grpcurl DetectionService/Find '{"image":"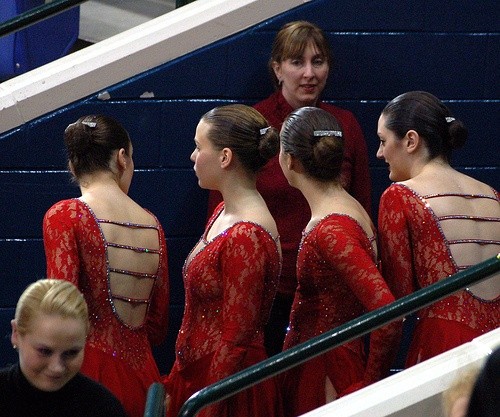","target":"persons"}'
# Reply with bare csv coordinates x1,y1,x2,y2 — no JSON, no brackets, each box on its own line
377,90,500,373
0,279,127,417
43,115,169,417
163,105,283,417
278,106,406,417
207,21,371,349
